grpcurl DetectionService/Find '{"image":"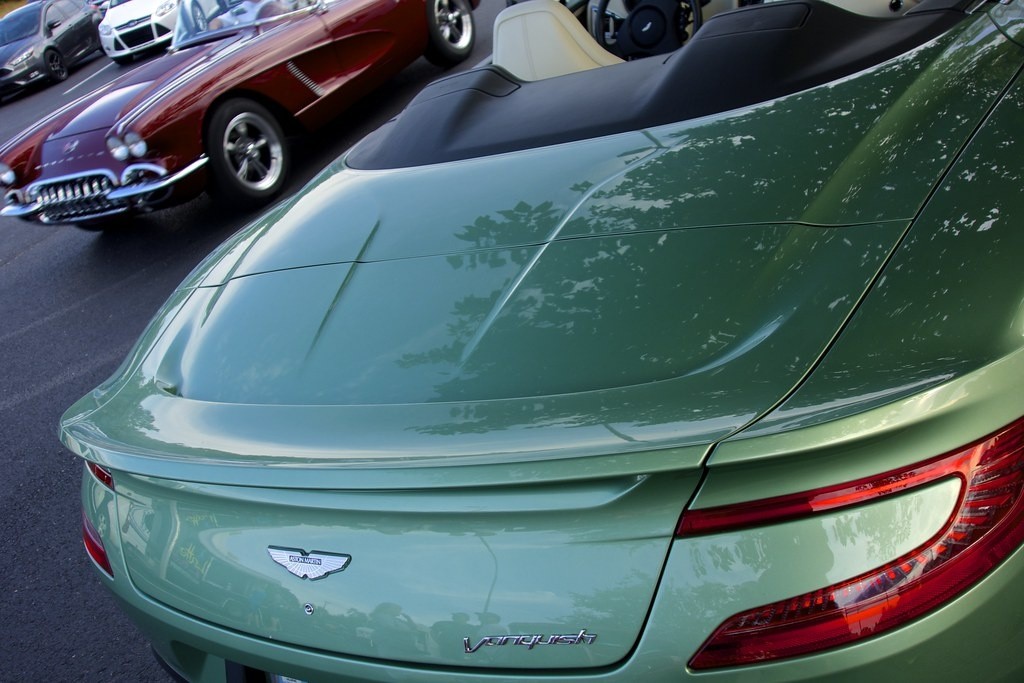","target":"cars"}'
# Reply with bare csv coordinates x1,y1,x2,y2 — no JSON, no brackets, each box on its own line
0,0,479,234
97,0,223,68
54,0,1021,681
0,0,103,102
